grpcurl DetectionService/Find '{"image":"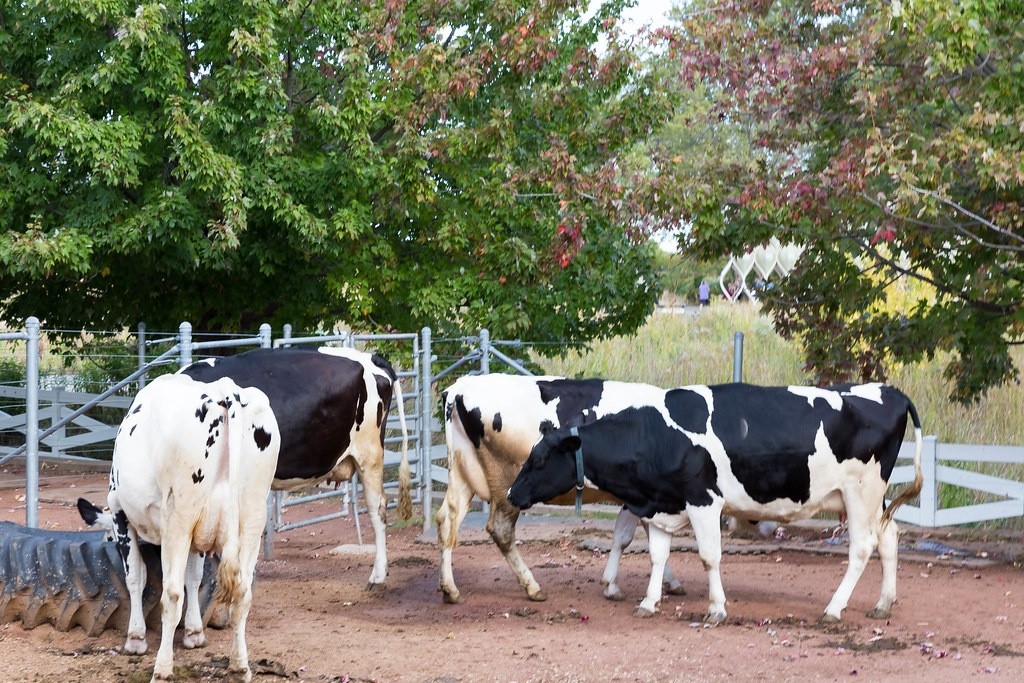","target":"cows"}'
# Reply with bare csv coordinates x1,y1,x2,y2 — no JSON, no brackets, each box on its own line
76,344,925,683
